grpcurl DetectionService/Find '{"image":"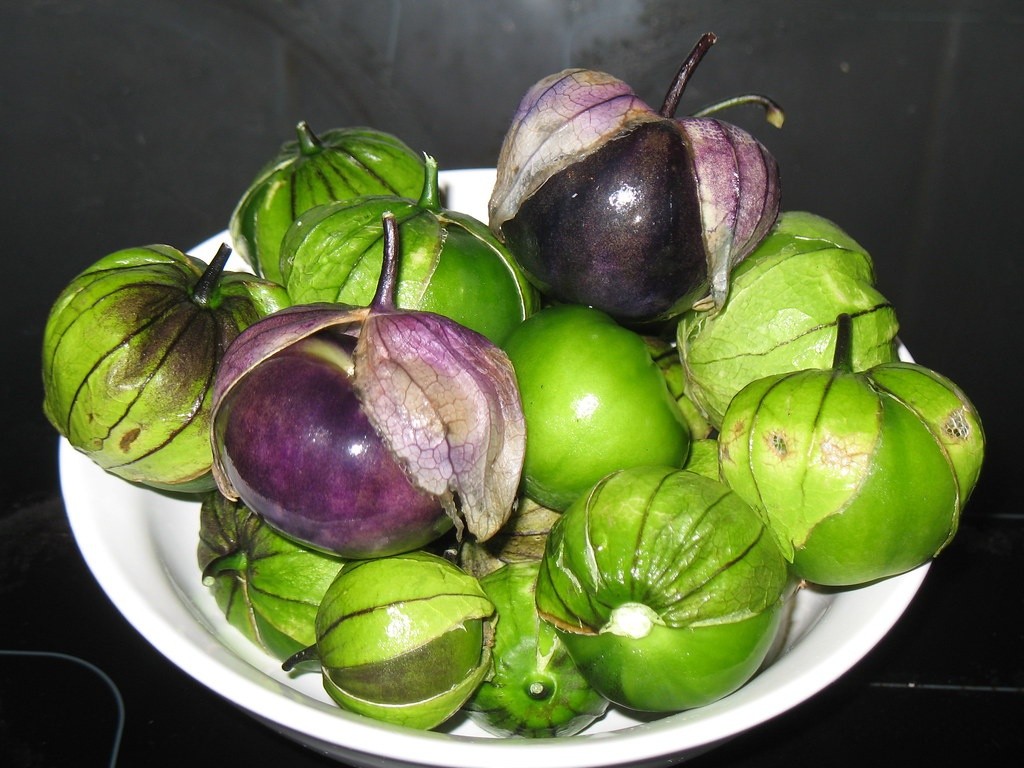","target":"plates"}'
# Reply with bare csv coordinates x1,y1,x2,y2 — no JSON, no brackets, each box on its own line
57,169,932,768
